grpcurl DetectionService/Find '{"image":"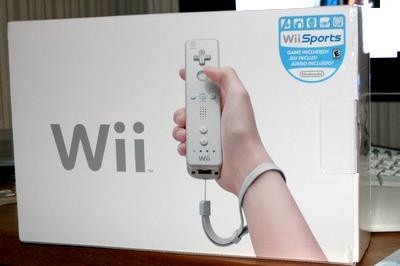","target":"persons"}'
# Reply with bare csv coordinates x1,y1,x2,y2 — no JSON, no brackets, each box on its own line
172,65,329,262
